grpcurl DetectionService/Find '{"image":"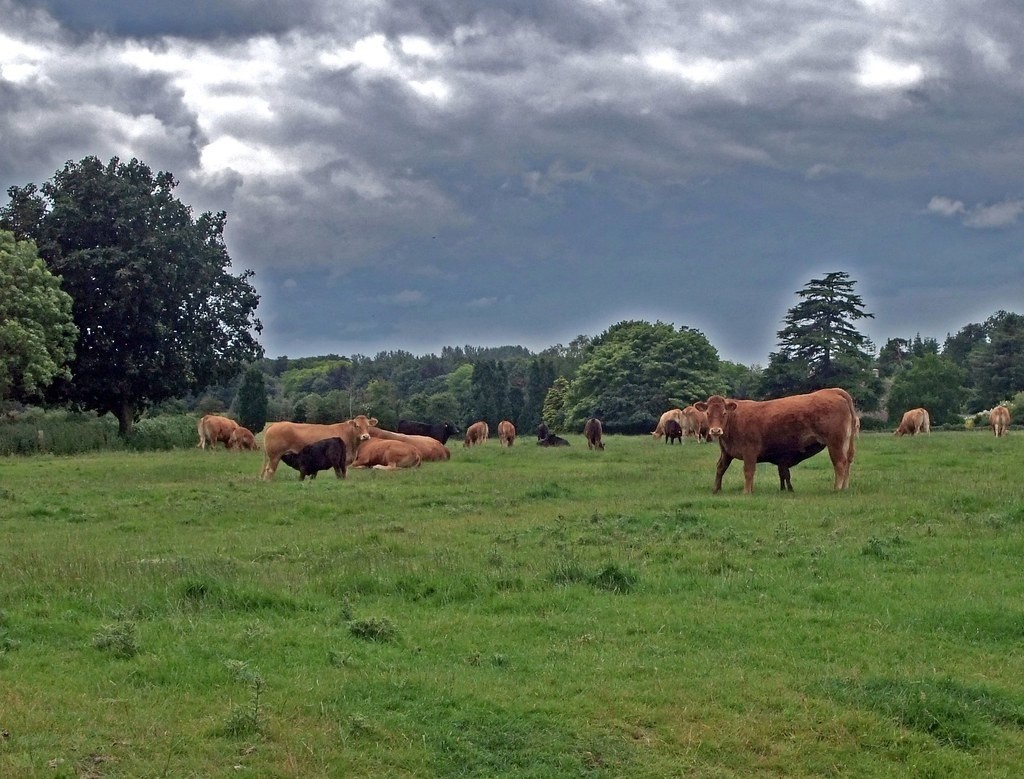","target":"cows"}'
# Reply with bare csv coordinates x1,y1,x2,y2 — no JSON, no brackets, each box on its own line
396,421,460,446
462,421,489,448
649,401,714,445
990,405,1011,436
692,388,860,494
348,426,450,471
894,407,930,437
536,423,571,448
258,414,378,481
497,420,516,446
197,414,260,451
584,418,606,451
